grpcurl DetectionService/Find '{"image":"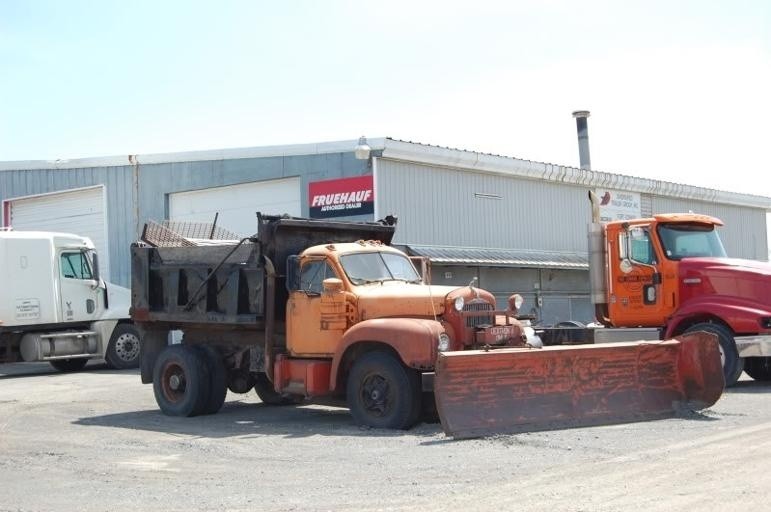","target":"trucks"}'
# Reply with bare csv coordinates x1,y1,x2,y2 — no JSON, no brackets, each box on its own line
516,189,771,390
